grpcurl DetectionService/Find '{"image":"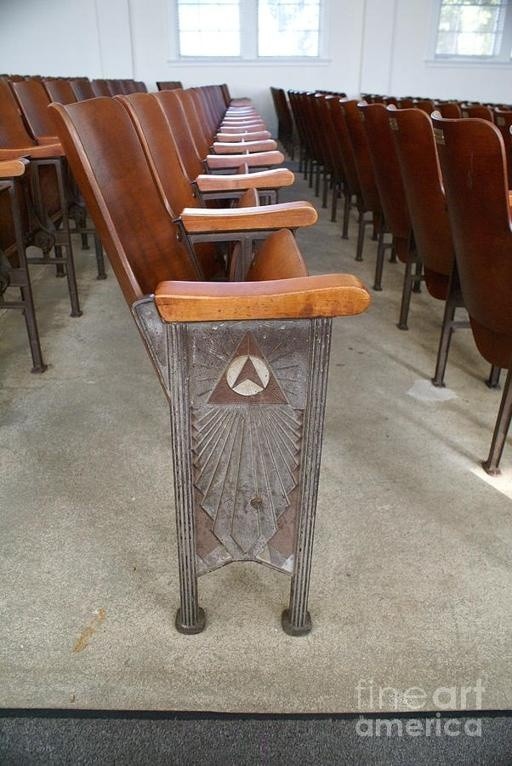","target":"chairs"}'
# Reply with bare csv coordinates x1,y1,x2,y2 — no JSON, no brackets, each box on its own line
0,72,511,637
